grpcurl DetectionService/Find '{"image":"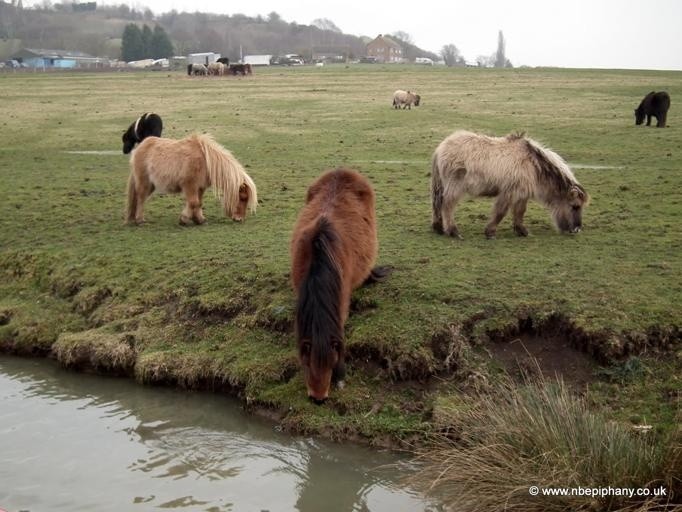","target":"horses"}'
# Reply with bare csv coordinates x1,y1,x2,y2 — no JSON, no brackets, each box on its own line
429,128,590,241
186,56,253,78
289,166,379,406
123,132,258,227
392,91,421,110
121,111,164,154
633,90,671,128
